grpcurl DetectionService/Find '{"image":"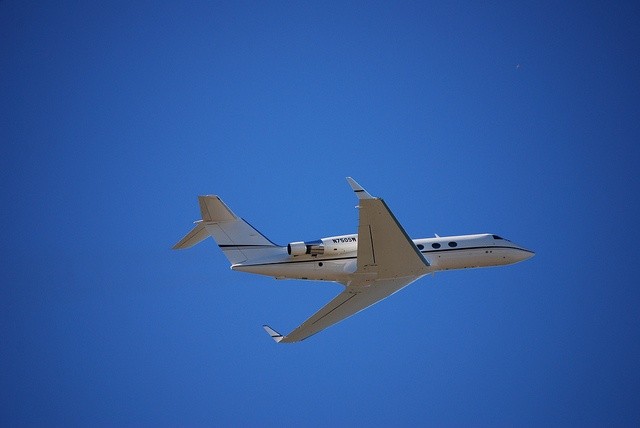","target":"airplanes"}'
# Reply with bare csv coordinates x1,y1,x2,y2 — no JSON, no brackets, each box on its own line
173,176,536,344
